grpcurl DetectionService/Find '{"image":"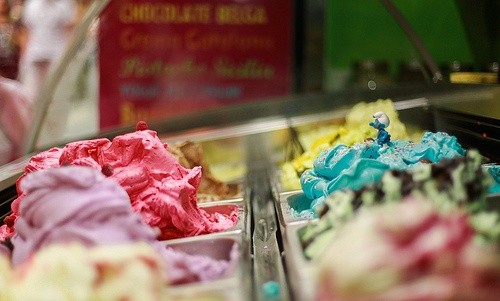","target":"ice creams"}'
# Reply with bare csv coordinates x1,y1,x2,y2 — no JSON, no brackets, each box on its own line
293,100,500,301
0,121,236,301
